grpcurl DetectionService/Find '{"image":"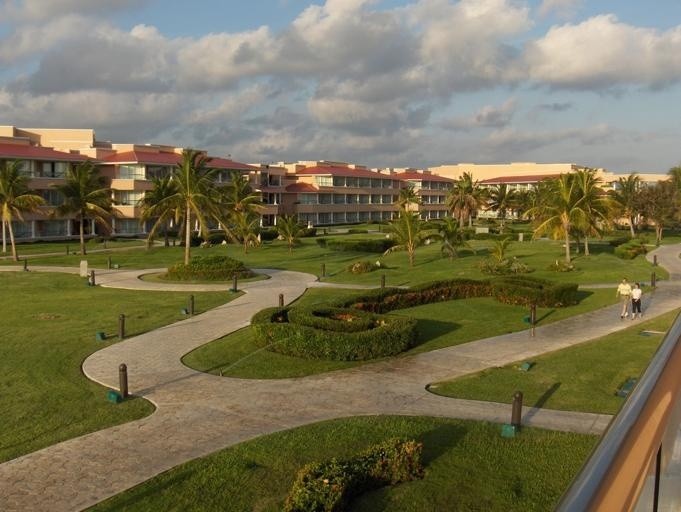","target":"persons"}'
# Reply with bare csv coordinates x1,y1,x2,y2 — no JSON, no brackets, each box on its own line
615,277,631,318
630,282,644,322
